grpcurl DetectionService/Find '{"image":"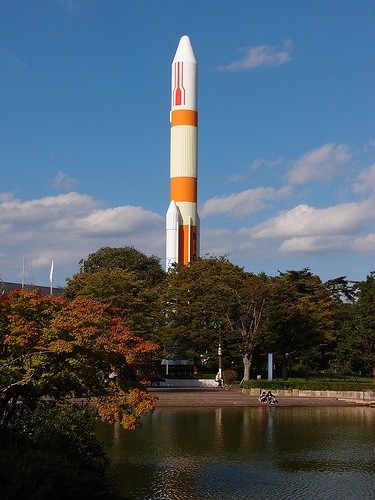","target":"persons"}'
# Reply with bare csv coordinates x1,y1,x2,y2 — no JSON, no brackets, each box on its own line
215,372,223,390
266,391,278,404
258,391,267,404
